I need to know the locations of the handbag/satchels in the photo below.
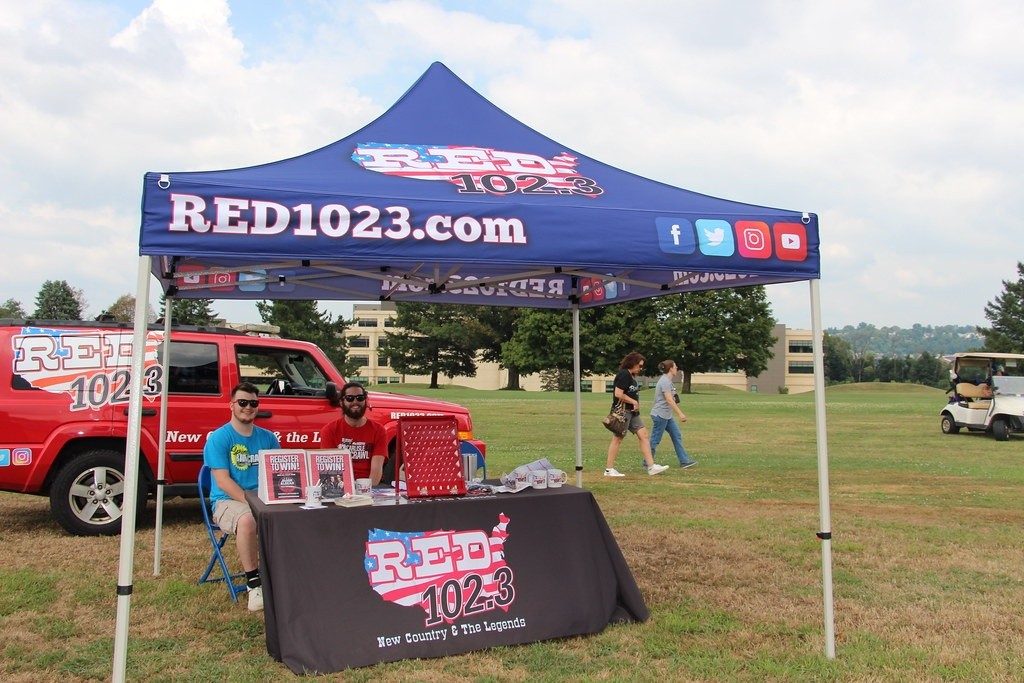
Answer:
[674,394,681,404]
[602,394,633,438]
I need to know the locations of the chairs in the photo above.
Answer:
[458,439,486,482]
[956,383,991,408]
[198,462,263,603]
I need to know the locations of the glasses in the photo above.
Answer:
[233,399,259,408]
[638,364,643,368]
[343,395,366,402]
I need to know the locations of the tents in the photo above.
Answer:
[112,60,836,683]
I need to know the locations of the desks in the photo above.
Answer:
[246,479,650,676]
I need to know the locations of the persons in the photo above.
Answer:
[203,384,281,612]
[327,474,344,492]
[604,352,669,476]
[320,382,390,487]
[642,360,698,469]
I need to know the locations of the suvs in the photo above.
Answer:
[0,318,488,538]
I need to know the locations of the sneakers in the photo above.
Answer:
[648,464,669,476]
[604,469,625,477]
[247,585,264,611]
[681,461,698,469]
[643,465,648,468]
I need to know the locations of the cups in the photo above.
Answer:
[548,469,567,488]
[304,486,322,507]
[355,478,372,496]
[462,453,477,483]
[527,469,547,489]
